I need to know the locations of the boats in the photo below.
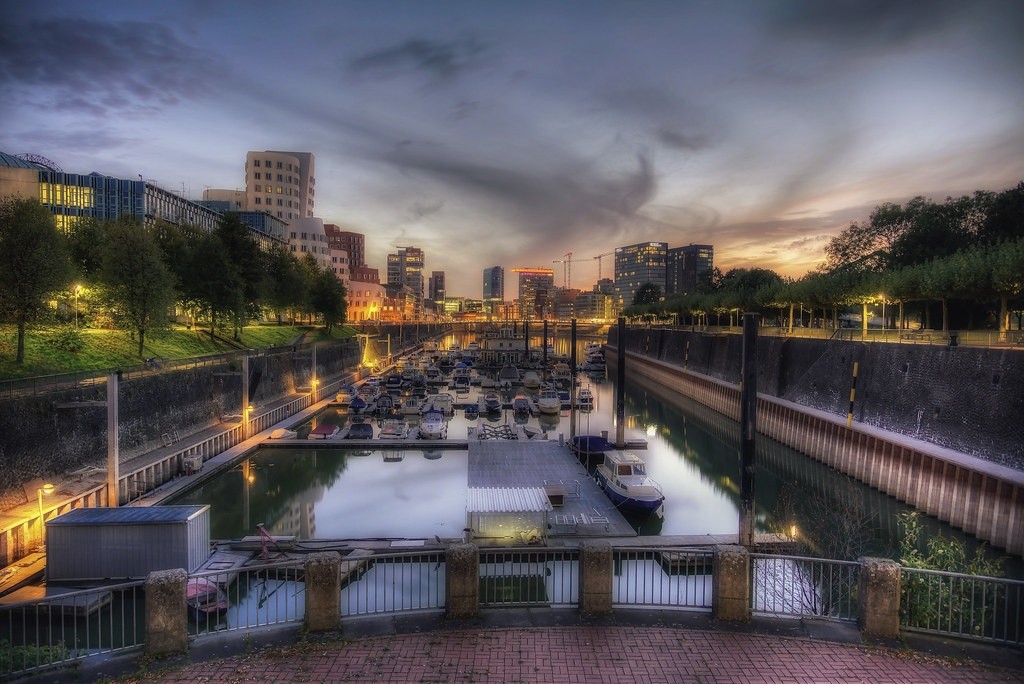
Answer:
[567,435,615,464]
[583,352,607,371]
[307,424,340,439]
[594,449,664,516]
[334,342,594,415]
[417,410,448,439]
[378,420,408,440]
[348,423,374,438]
[270,428,297,440]
[583,344,601,356]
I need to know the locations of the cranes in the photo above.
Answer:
[552,252,595,290]
[593,250,615,280]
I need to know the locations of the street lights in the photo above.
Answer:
[74,284,82,330]
[879,296,885,337]
